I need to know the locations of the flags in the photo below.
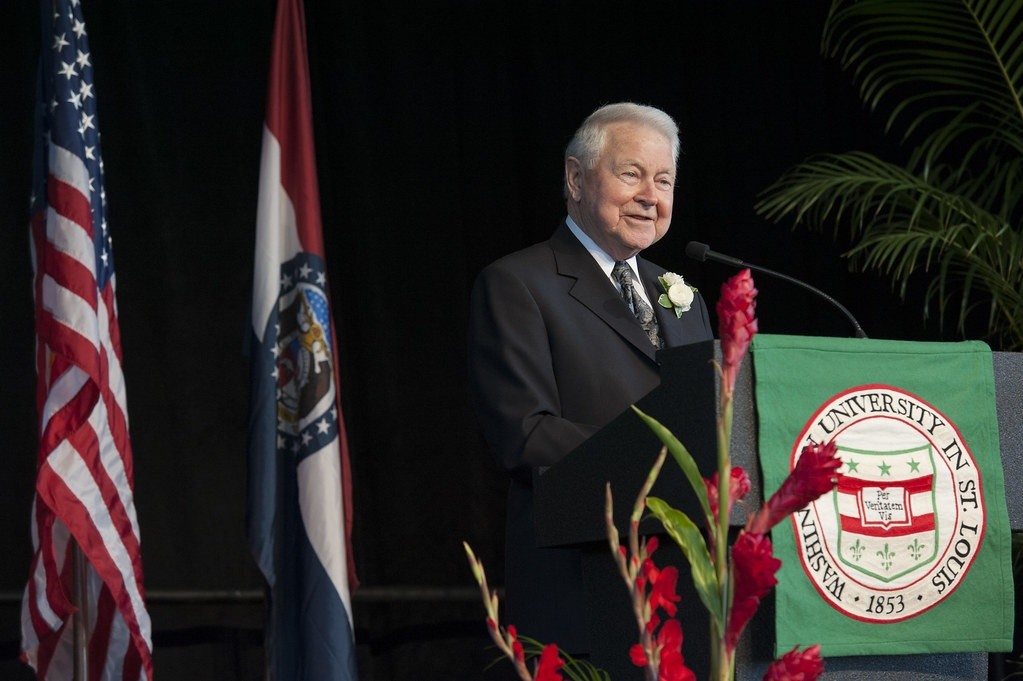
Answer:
[240,0,359,680]
[20,0,153,680]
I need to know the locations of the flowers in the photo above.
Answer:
[461,269,844,681]
[655,271,700,318]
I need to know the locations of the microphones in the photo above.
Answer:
[683,241,870,339]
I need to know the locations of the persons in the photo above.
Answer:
[464,102,714,655]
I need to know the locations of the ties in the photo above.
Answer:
[610,259,666,351]
